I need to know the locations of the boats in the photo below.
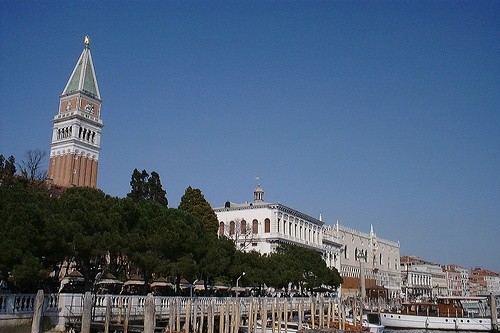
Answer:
[381,301,500,332]
[240,320,385,333]
